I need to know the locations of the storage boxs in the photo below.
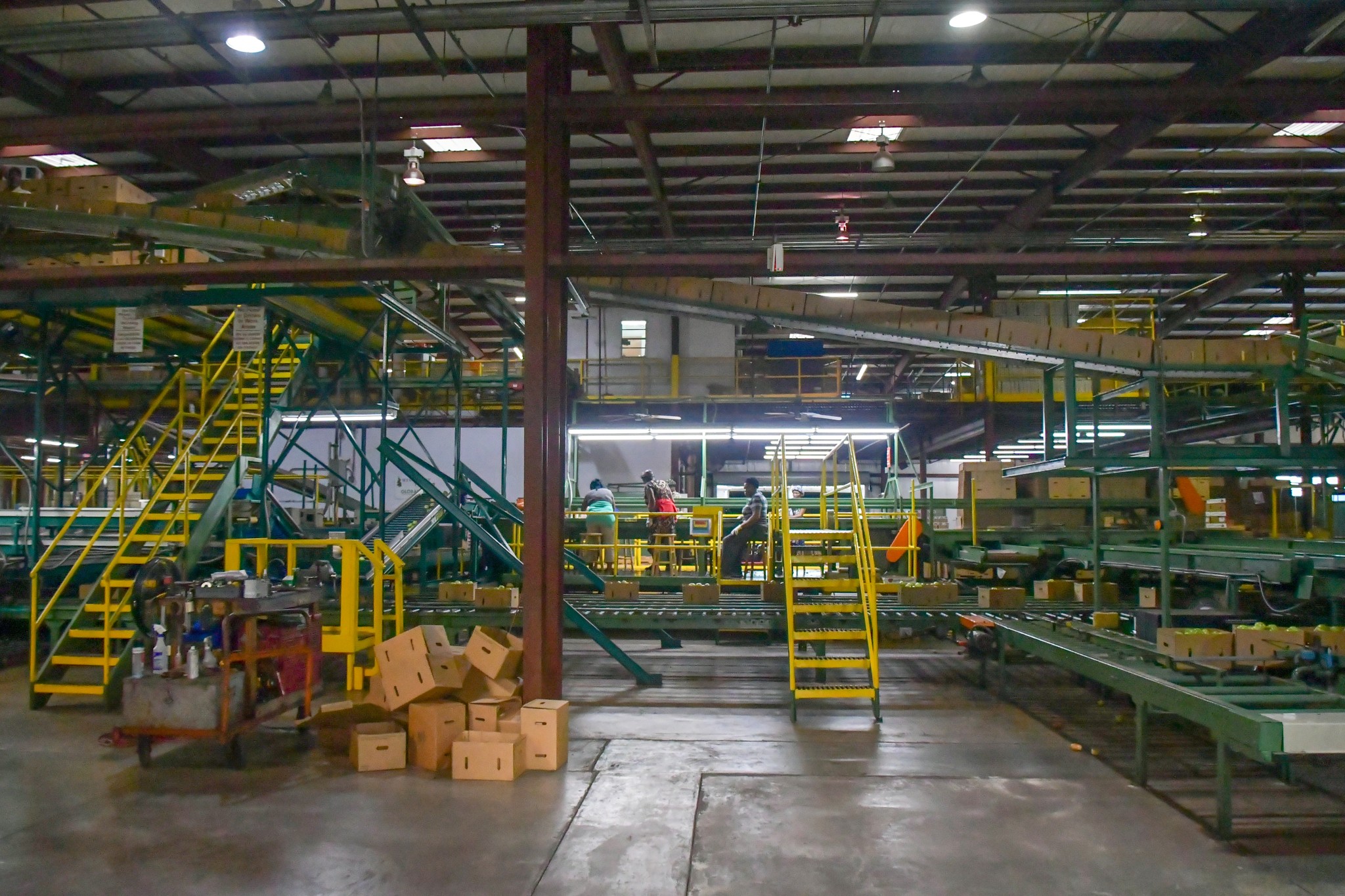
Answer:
[438,580,477,602]
[1299,626,1345,656]
[682,582,719,604]
[761,581,797,602]
[1233,625,1304,666]
[474,586,519,612]
[955,461,1225,532]
[421,238,1155,401]
[891,577,1190,628]
[319,624,568,780]
[124,670,247,726]
[1154,335,1292,381]
[604,580,640,602]
[1157,625,1233,670]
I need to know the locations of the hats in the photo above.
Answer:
[745,477,759,488]
[792,484,804,496]
[640,470,655,478]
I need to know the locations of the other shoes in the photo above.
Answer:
[716,572,743,579]
[645,564,659,571]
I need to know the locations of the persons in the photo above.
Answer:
[581,478,618,573]
[710,476,769,581]
[640,469,677,575]
[775,484,808,575]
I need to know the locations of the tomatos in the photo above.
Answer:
[770,580,776,584]
[407,490,448,532]
[991,587,997,590]
[1172,622,1345,634]
[610,580,633,584]
[450,579,512,590]
[1070,699,1124,755]
[1041,579,1054,582]
[987,529,996,531]
[899,580,939,587]
[688,583,710,586]
[1066,620,1073,626]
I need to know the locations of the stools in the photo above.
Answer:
[744,538,766,579]
[650,532,678,576]
[615,539,634,571]
[579,532,604,573]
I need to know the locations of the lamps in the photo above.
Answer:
[872,128,896,172]
[402,140,426,186]
[835,200,850,239]
[1187,196,1209,236]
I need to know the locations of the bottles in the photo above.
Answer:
[132,647,146,679]
[186,646,200,679]
